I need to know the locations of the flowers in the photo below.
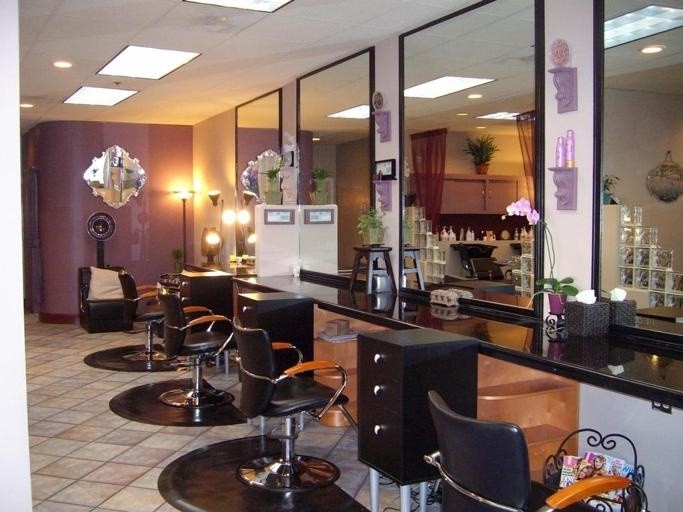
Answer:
[499,196,579,299]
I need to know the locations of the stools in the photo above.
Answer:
[402,246,425,291]
[348,244,390,298]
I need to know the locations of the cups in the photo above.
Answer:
[264,191,282,205]
[486,229,494,241]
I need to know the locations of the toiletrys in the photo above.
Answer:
[566,130,575,168]
[434,225,534,242]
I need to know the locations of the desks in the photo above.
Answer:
[176,254,683,413]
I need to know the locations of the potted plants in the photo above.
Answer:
[356,207,387,247]
[600,171,620,206]
[464,132,500,175]
[308,167,333,205]
[262,165,283,205]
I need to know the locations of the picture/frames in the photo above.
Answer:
[263,207,296,227]
[302,207,335,226]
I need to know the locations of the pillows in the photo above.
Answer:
[82,264,126,302]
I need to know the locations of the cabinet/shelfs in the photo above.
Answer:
[235,292,314,436]
[617,204,681,310]
[405,205,447,287]
[355,326,481,512]
[426,173,522,216]
[510,228,534,297]
[177,265,228,374]
[476,350,583,490]
[308,301,393,429]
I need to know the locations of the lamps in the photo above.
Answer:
[200,226,223,268]
[239,188,256,208]
[169,178,195,263]
[205,187,232,269]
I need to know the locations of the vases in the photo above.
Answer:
[545,291,569,316]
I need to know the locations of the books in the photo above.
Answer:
[316,329,359,343]
[558,451,634,511]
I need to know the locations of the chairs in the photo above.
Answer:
[467,256,509,279]
[117,268,178,362]
[151,281,236,413]
[75,266,130,335]
[226,314,357,495]
[420,389,651,511]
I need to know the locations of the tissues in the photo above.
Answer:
[564,289,610,338]
[600,288,637,327]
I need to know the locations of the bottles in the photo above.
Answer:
[513,226,518,240]
[564,129,574,168]
[519,226,533,240]
[440,225,485,240]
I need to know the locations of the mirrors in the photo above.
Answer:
[397,0,549,321]
[594,0,682,352]
[295,44,377,292]
[232,83,285,271]
[80,143,149,210]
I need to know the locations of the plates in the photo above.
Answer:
[501,230,509,239]
